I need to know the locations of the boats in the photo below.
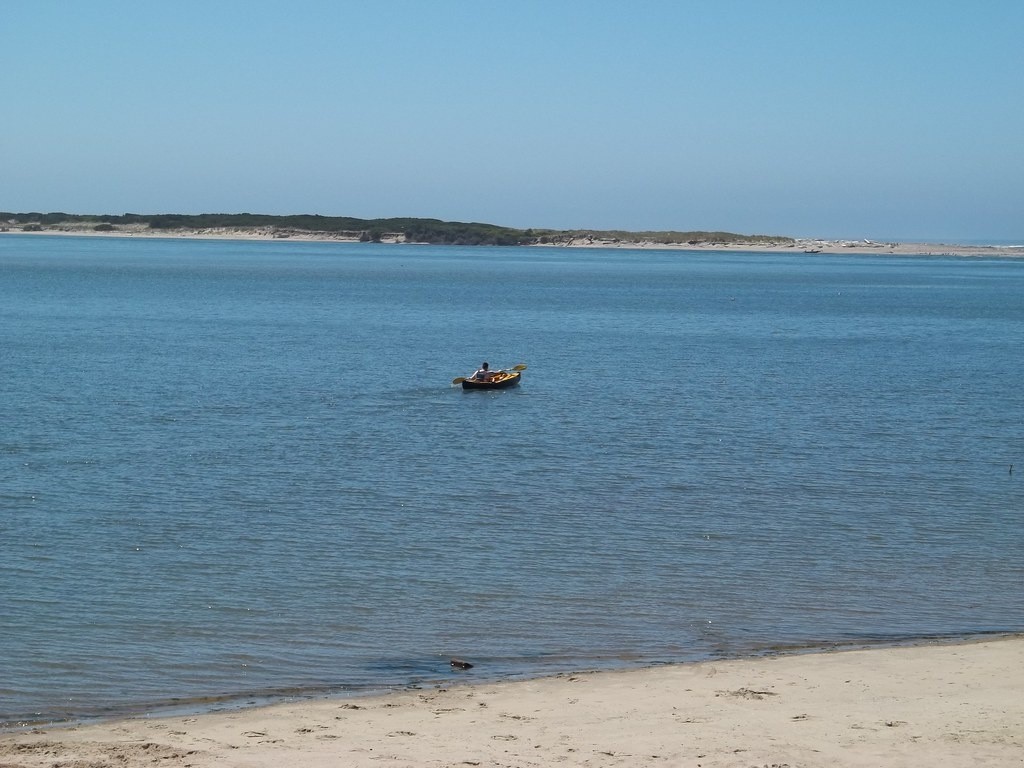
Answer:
[461,371,522,391]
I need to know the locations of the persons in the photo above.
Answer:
[467,362,504,382]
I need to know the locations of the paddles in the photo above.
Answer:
[452,364,527,385]
